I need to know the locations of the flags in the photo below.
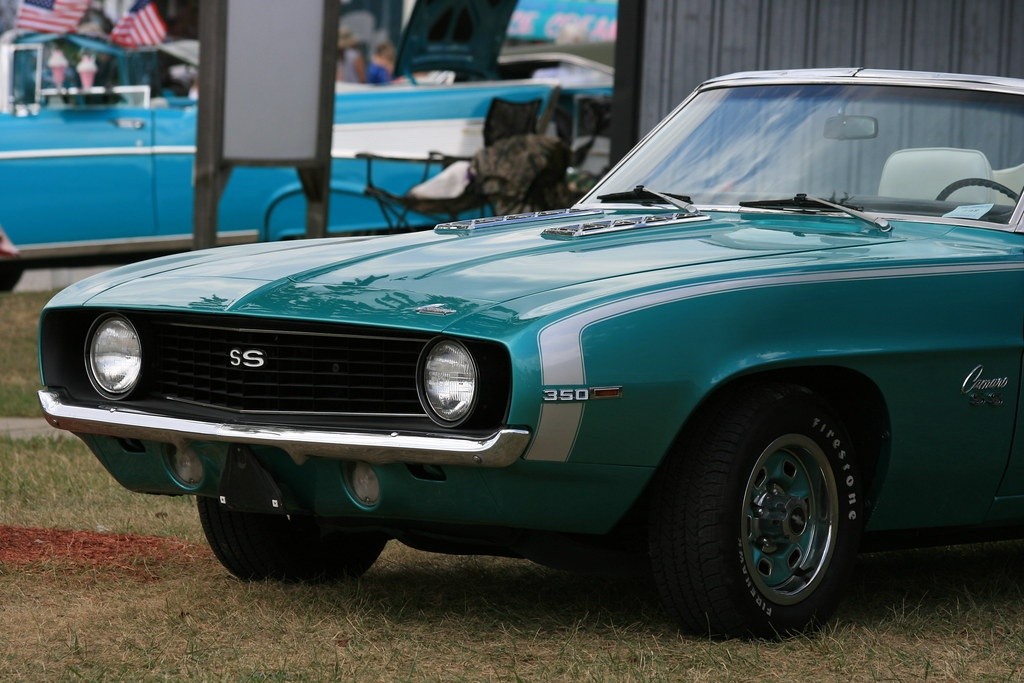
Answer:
[13,0,168,49]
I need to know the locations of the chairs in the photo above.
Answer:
[879,147,996,205]
[354,84,618,232]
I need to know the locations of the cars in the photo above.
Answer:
[35,64,1024,638]
[0,0,615,290]
